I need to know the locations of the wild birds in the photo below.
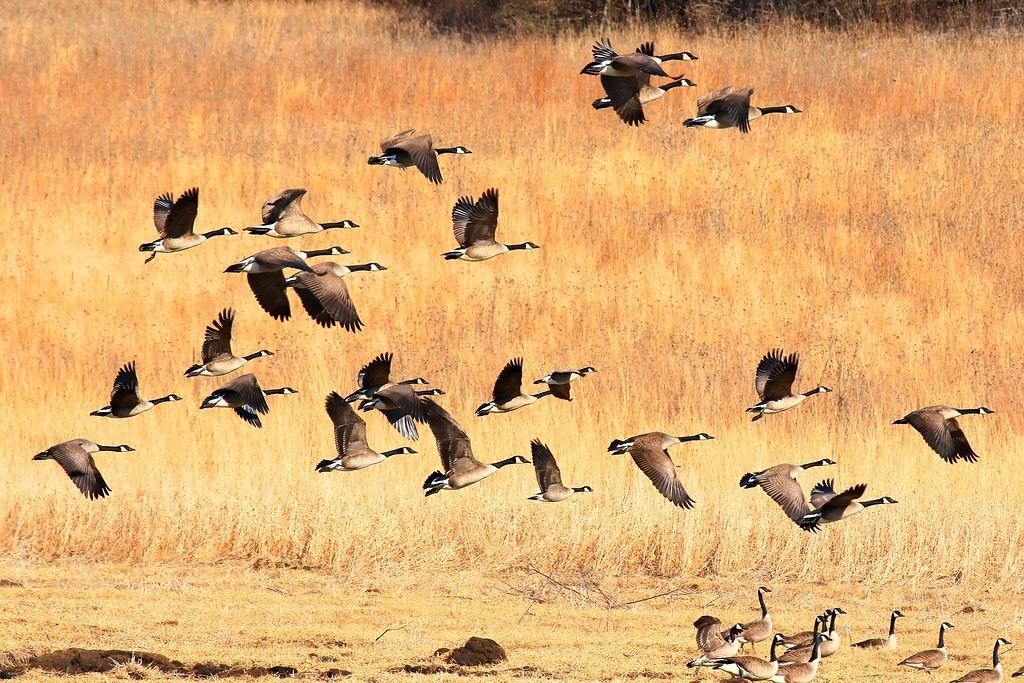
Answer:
[607,433,716,510]
[242,188,361,237]
[313,390,418,473]
[475,357,598,416]
[286,262,387,333]
[744,347,835,424]
[31,438,136,501]
[682,87,802,134]
[739,405,994,534]
[138,186,238,264]
[579,36,699,126]
[342,350,532,497]
[366,130,472,185]
[199,373,299,428]
[182,307,275,378]
[89,361,183,418]
[686,586,1024,683]
[438,188,541,262]
[528,438,594,502]
[223,245,352,322]
